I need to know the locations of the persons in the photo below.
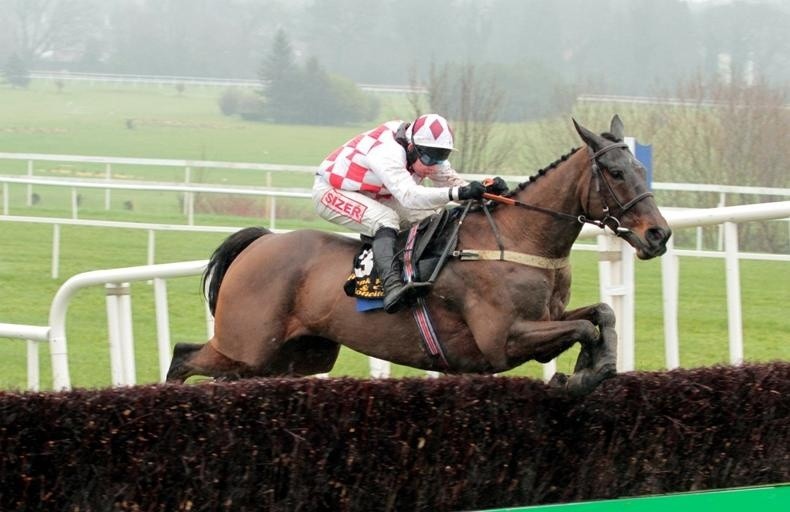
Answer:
[307,113,508,313]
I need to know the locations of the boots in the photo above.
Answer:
[370,229,434,315]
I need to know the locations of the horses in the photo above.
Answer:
[165,114,673,383]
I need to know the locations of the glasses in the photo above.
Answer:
[411,118,446,166]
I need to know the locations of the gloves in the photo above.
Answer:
[457,179,487,202]
[481,177,510,195]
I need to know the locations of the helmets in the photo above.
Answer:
[403,114,463,152]
